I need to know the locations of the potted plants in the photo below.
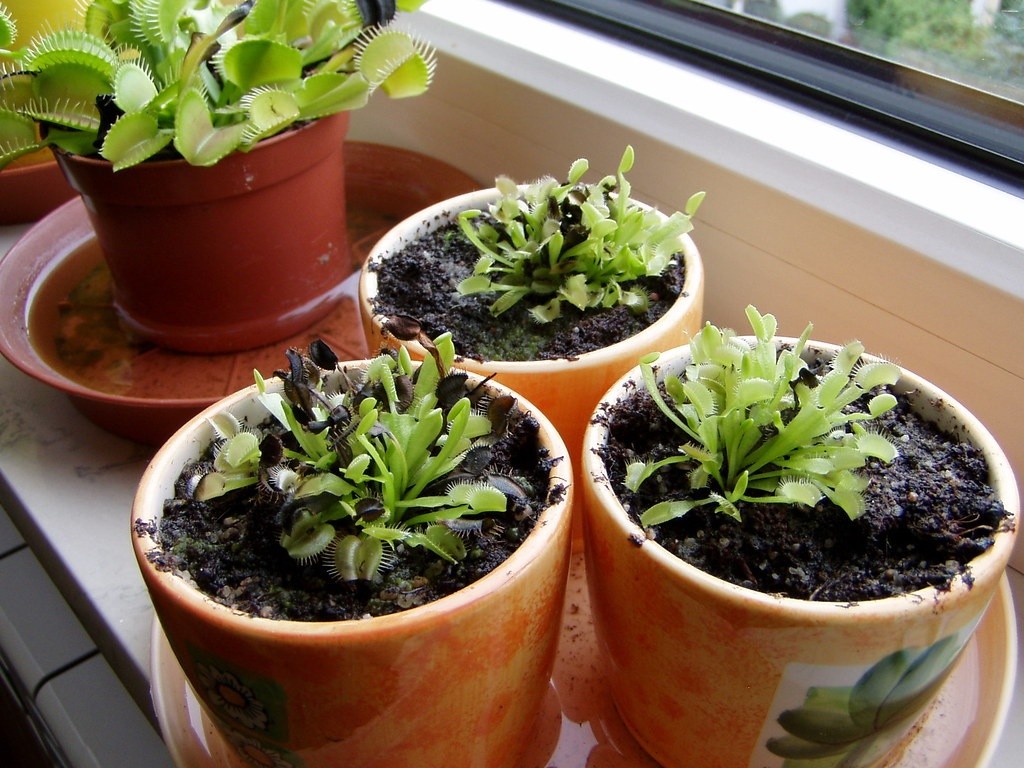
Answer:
[0,0,438,354]
[130,330,575,768]
[581,302,1021,768]
[359,144,706,541]
